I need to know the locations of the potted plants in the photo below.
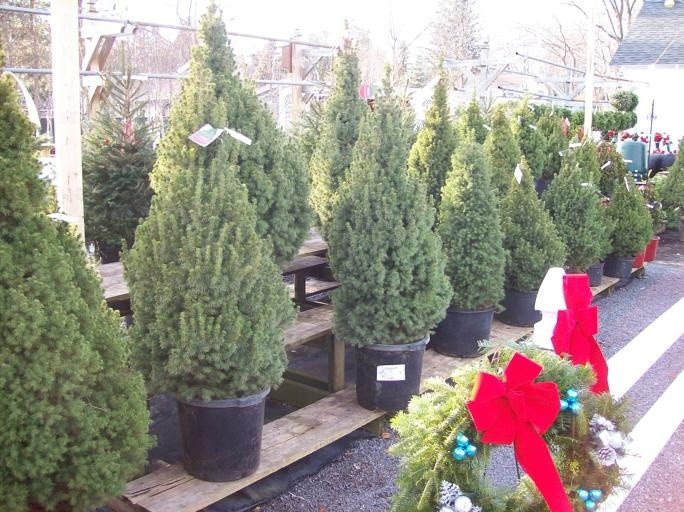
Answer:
[127,71,299,485]
[328,78,450,415]
[436,107,677,360]
